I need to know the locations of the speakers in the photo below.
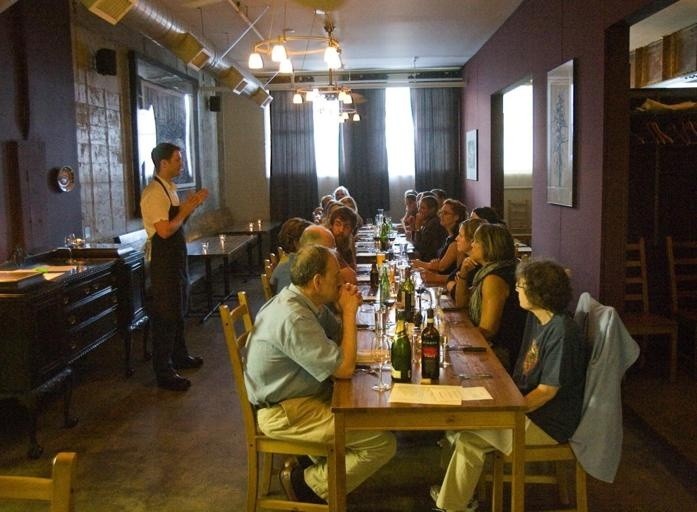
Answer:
[210,96,220,111]
[96,48,117,75]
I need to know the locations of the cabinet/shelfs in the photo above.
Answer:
[0,239,153,462]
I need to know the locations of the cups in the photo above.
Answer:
[219,233,227,245]
[200,241,209,255]
[248,218,263,231]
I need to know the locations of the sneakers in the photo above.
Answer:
[177,353,203,367]
[159,371,191,390]
[427,482,484,510]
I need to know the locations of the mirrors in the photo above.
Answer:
[126,47,203,220]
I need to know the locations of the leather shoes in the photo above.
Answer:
[278,456,302,512]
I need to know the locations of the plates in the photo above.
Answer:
[57,166,76,193]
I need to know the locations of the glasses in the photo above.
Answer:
[436,208,459,218]
[514,279,529,292]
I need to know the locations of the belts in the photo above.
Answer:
[251,399,290,412]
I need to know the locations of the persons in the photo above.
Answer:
[139,142,209,392]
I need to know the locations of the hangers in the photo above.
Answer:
[627,106,696,150]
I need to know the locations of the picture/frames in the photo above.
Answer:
[464,129,479,182]
[544,56,579,210]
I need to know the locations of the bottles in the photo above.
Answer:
[371,209,441,389]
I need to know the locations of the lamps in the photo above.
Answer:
[245,0,361,126]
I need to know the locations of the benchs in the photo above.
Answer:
[112,205,265,326]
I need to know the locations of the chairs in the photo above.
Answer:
[219,291,336,512]
[262,246,287,302]
[505,199,531,250]
[617,233,680,387]
[0,451,79,512]
[663,235,696,375]
[478,292,616,512]
